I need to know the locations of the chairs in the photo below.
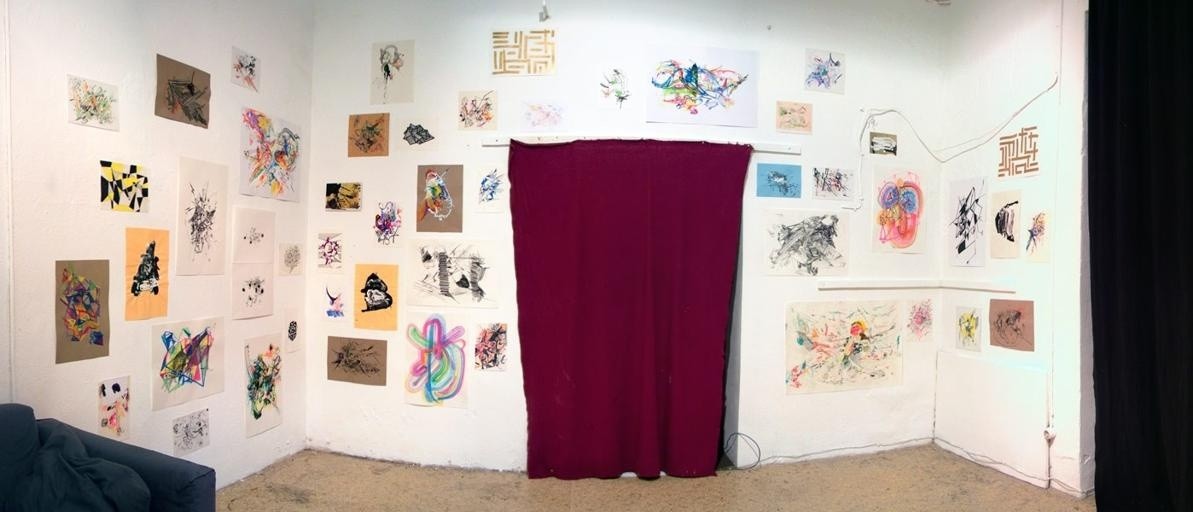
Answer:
[0,401,216,511]
